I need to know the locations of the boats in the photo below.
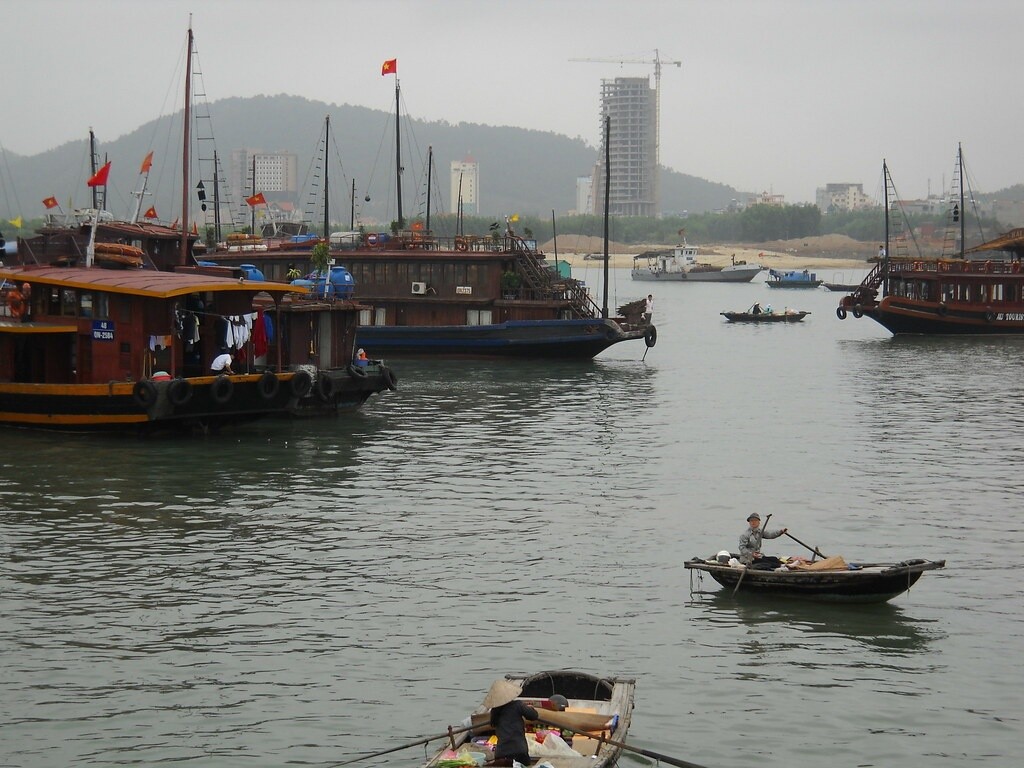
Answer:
[836,228,1024,332]
[0,220,396,435]
[682,551,945,604]
[719,309,811,323]
[825,282,865,290]
[631,245,765,282]
[766,267,820,289]
[186,230,659,358]
[418,668,636,768]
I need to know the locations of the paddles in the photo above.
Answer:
[759,306,765,312]
[537,717,709,768]
[747,301,757,313]
[324,720,491,768]
[732,514,772,600]
[784,528,827,559]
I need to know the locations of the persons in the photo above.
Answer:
[210,348,237,375]
[287,262,302,279]
[738,512,788,568]
[753,302,762,314]
[644,294,654,323]
[0,232,7,263]
[150,344,172,376]
[765,303,775,314]
[878,245,885,256]
[490,681,538,767]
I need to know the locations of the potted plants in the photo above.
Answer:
[286,268,301,304]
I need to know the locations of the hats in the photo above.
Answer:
[747,513,761,523]
[483,679,523,708]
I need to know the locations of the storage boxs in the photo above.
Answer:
[352,359,368,367]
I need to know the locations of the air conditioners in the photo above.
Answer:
[411,282,427,294]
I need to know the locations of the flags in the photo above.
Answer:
[381,58,397,76]
[87,162,111,186]
[43,197,58,209]
[509,213,519,223]
[9,217,21,228]
[411,223,423,230]
[144,207,159,219]
[191,221,199,234]
[139,153,153,174]
[244,192,266,206]
[679,228,685,236]
[171,217,178,230]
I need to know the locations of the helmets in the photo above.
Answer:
[717,550,731,564]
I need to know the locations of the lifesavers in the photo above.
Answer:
[454,239,468,251]
[836,304,864,320]
[347,364,398,391]
[656,273,659,277]
[606,326,657,347]
[6,292,26,317]
[258,371,333,404]
[682,273,686,278]
[937,304,997,322]
[132,377,234,407]
[912,261,1022,275]
[365,233,380,247]
[226,234,264,245]
[92,243,144,265]
